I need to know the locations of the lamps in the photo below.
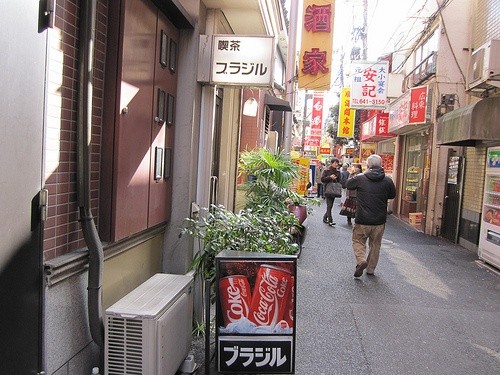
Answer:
[244,86,258,117]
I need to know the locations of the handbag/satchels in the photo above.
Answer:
[325,179,342,198]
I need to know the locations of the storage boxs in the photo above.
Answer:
[408,212,422,225]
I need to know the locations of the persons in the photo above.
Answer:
[316,160,362,226]
[346,155,397,277]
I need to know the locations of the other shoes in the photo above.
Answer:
[366,270,374,275]
[354,261,368,277]
[323,218,329,223]
[329,221,336,225]
[348,219,352,225]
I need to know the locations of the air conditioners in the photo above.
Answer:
[103,272,195,375]
[466,39,500,92]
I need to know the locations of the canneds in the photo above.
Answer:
[284,276,294,328]
[248,264,291,327]
[219,275,252,328]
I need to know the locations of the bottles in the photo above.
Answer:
[486,176,500,208]
